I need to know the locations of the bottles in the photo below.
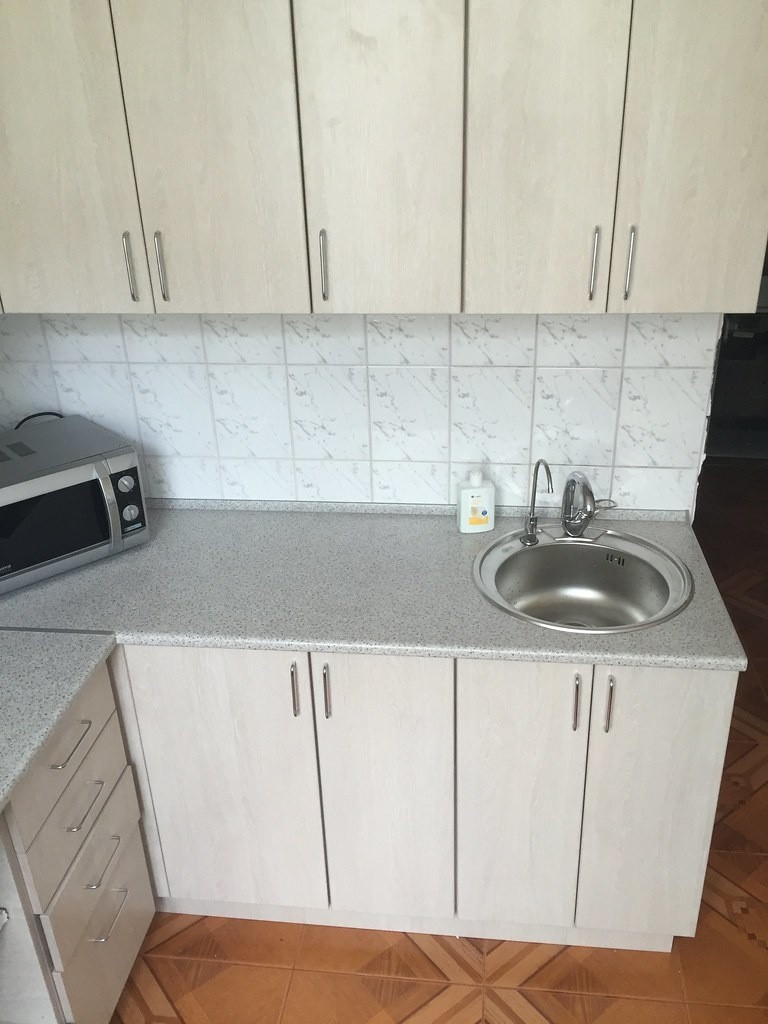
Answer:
[457,469,496,533]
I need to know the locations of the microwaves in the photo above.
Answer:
[0,414,150,594]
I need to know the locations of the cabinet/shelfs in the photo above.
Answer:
[462,0,768,315]
[291,0,466,316]
[456,657,740,953]
[0,0,315,316]
[122,643,458,936]
[0,663,156,1024]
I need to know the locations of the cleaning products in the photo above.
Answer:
[455,469,496,534]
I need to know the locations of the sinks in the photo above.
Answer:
[472,518,696,635]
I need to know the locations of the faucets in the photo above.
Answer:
[561,472,596,538]
[526,458,553,534]
